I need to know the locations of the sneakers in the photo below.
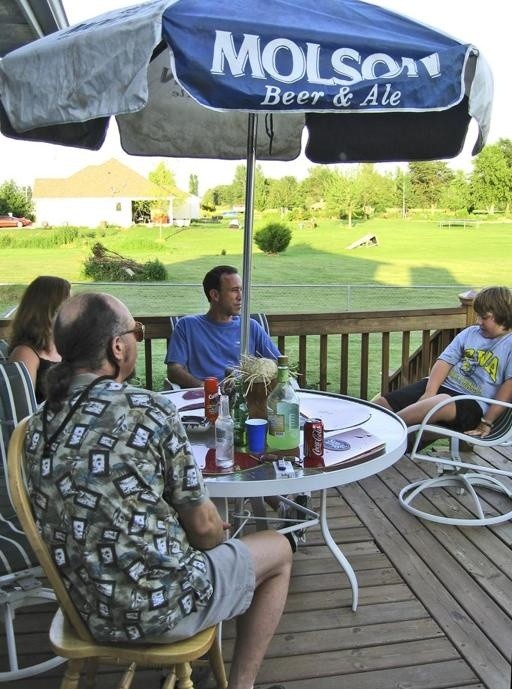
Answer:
[275,525,307,552]
[286,491,311,528]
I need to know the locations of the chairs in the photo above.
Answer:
[163,313,299,393]
[398,380,512,526]
[5,413,226,689]
[1,336,71,682]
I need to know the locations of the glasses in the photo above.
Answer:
[119,321,147,343]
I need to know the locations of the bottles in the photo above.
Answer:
[213,394,236,467]
[262,353,300,451]
[230,379,252,453]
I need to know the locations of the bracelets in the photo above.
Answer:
[480,418,493,428]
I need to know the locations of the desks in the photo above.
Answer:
[159,387,410,652]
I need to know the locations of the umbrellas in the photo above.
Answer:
[0,0,494,530]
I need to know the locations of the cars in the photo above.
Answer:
[0,216,33,228]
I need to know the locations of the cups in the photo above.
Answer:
[245,419,269,454]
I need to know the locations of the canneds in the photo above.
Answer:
[204,377,218,422]
[303,419,324,459]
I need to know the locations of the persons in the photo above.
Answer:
[164,265,310,522]
[369,286,512,447]
[9,273,72,406]
[22,292,293,686]
